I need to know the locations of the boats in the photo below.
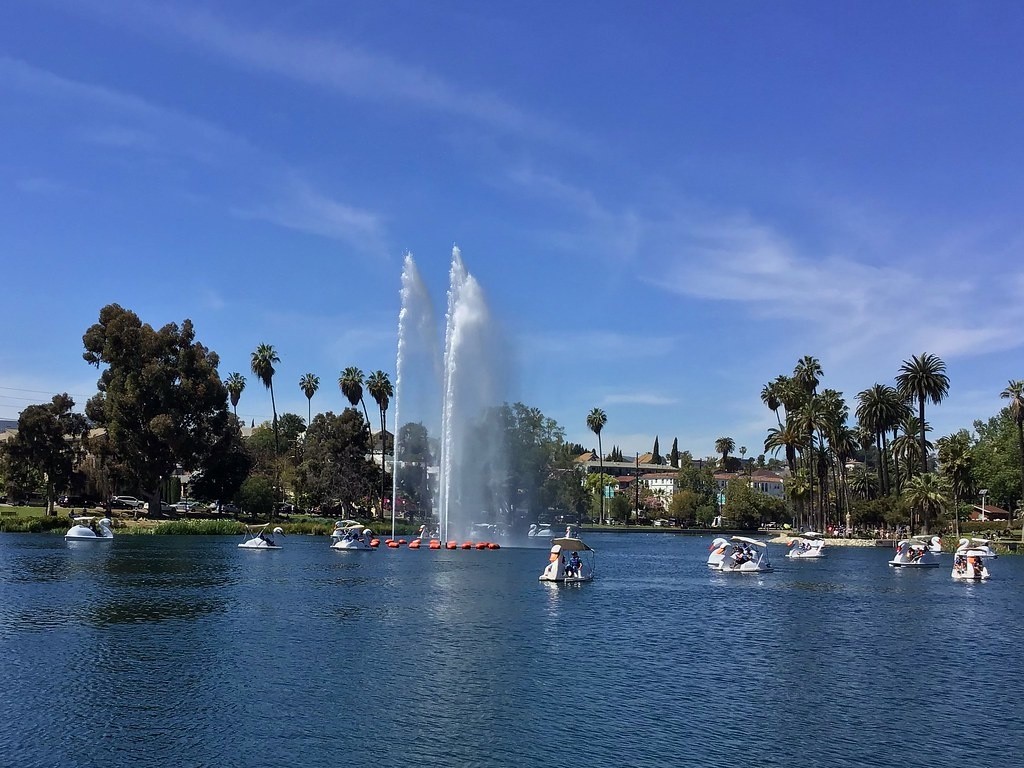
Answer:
[330,519,379,550]
[888,534,1000,579]
[528,522,557,537]
[64,515,114,539]
[237,522,283,549]
[416,520,454,539]
[707,535,775,572]
[785,531,829,558]
[538,537,596,581]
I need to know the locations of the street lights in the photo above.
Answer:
[978,488,988,522]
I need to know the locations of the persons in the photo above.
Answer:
[906,545,929,563]
[572,528,578,539]
[70,502,112,537]
[977,528,1011,540]
[953,555,983,576]
[729,542,753,569]
[800,523,907,540]
[343,529,359,541]
[938,531,942,538]
[958,524,963,536]
[260,533,274,545]
[561,551,583,578]
[800,542,811,554]
[64,495,69,508]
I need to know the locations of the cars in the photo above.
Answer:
[0,490,307,517]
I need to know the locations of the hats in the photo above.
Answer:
[572,552,577,555]
